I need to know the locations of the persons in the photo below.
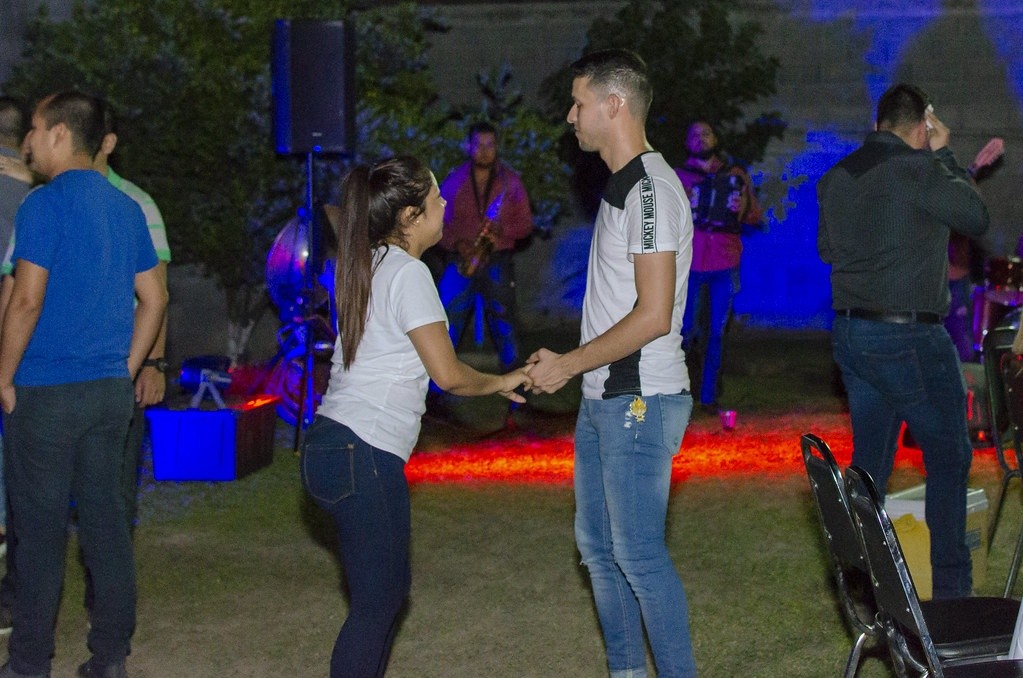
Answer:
[813,80,987,603]
[944,229,980,362]
[302,156,534,678]
[430,121,532,436]
[674,117,762,419]
[525,51,695,678]
[0,89,172,678]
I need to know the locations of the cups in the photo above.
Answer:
[719,410,737,431]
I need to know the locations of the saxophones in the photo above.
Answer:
[456,159,510,282]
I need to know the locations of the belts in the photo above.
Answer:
[838,306,939,325]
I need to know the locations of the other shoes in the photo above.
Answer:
[0,659,49,678]
[78,654,126,678]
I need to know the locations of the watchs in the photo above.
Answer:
[144,358,167,372]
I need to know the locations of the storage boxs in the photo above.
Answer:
[144,394,282,481]
[881,483,988,600]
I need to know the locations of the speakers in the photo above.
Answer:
[271,17,356,163]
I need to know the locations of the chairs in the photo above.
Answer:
[800,308,1023,677]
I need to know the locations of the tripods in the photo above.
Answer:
[254,157,332,458]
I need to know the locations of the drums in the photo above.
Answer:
[982,253,1023,306]
